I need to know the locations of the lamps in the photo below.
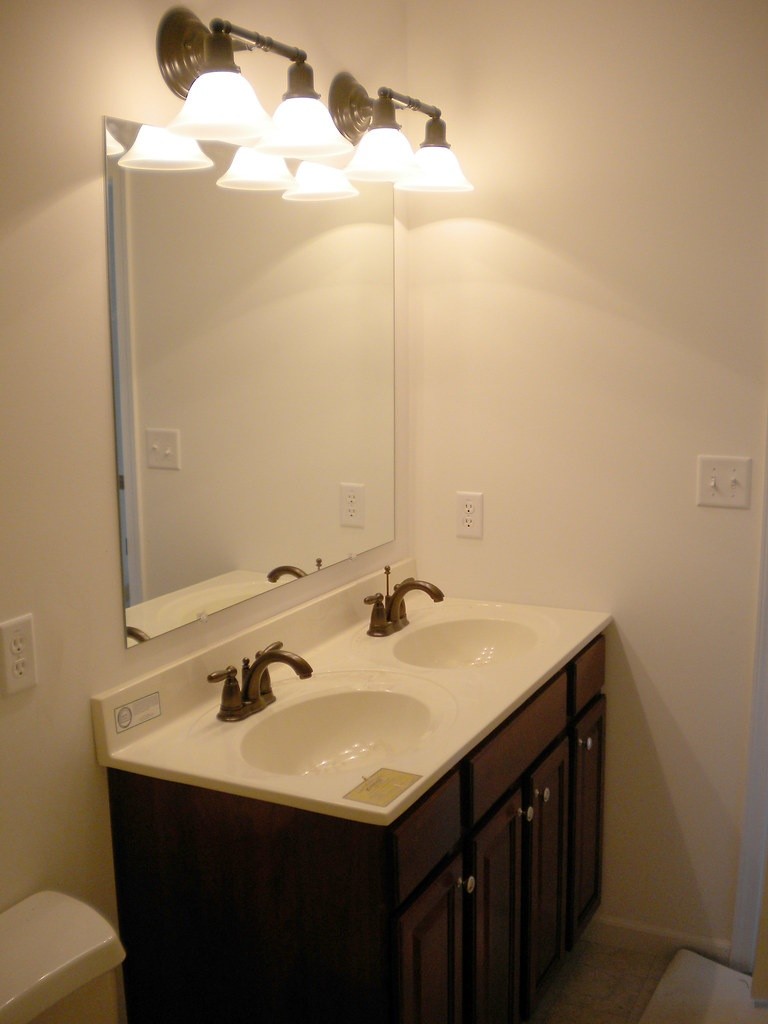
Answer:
[215,146,360,202]
[328,71,475,193]
[154,7,349,156]
[102,117,215,171]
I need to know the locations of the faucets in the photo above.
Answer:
[266,557,324,585]
[237,647,315,713]
[377,579,446,637]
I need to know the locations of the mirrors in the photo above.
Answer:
[99,109,400,652]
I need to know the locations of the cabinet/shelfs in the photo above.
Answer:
[108,630,614,1024]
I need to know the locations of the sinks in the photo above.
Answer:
[390,616,544,673]
[156,584,267,627]
[240,688,442,787]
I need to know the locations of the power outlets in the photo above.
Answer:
[1,613,39,694]
